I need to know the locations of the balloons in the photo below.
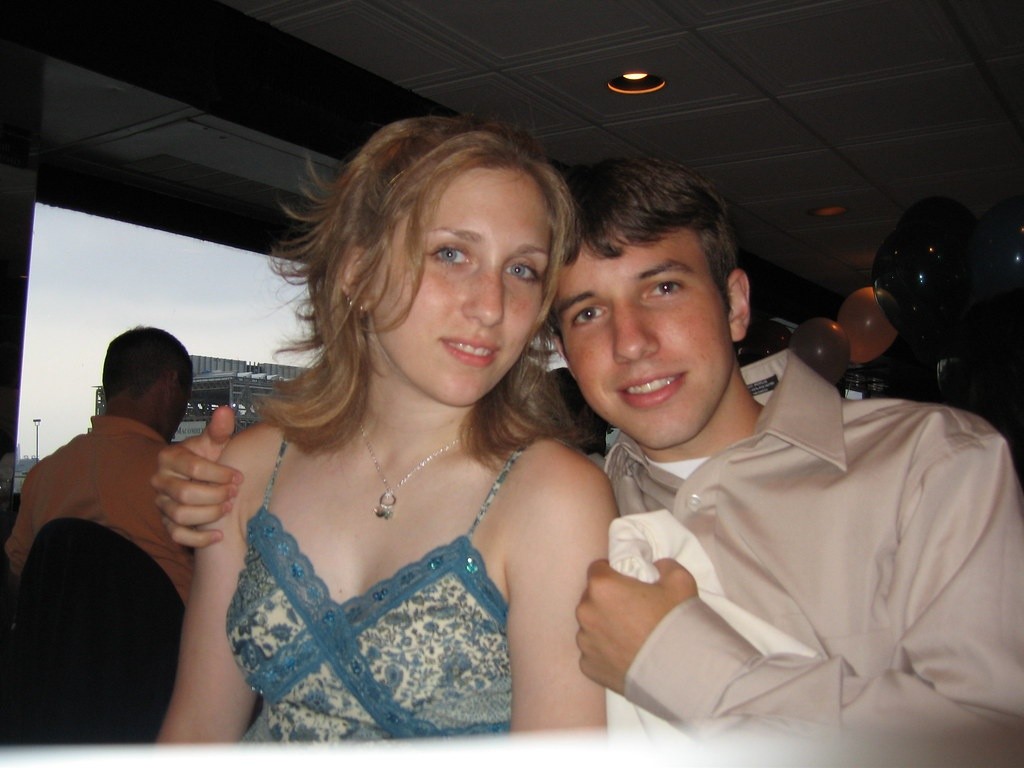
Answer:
[738,195,1024,386]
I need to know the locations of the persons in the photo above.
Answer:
[148,155,1024,757]
[3,328,194,606]
[542,368,612,460]
[156,116,618,745]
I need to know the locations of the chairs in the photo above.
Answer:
[0,516,185,744]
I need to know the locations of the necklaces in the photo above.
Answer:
[359,418,468,520]
